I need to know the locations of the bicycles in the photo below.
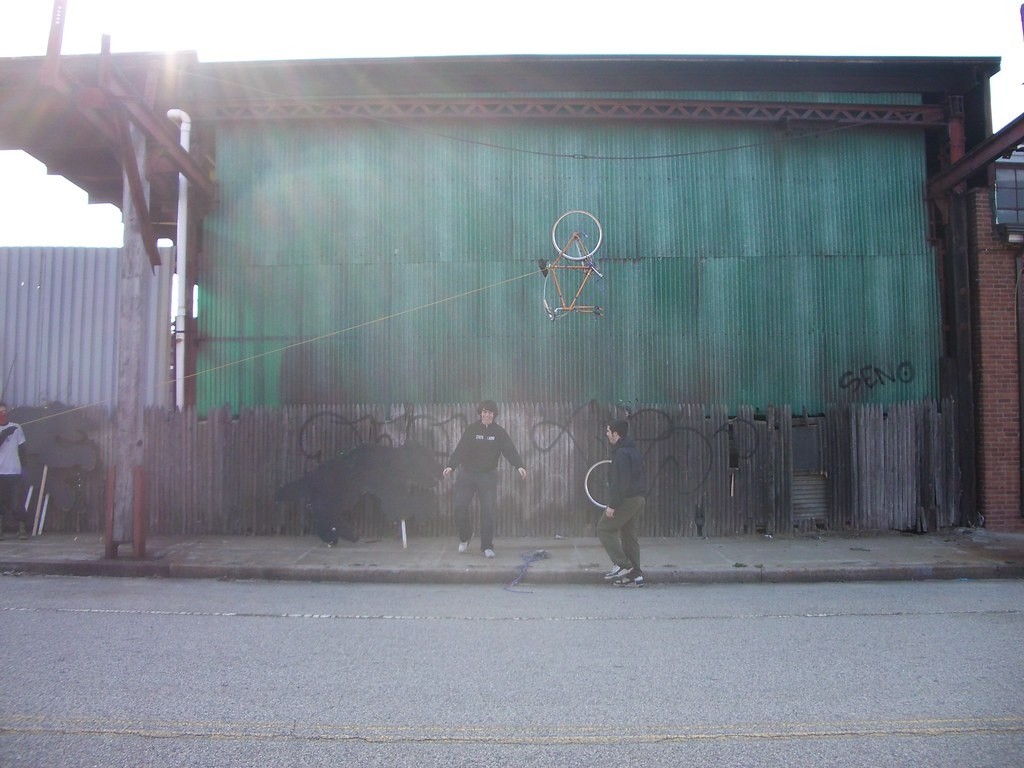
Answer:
[584,459,612,509]
[542,209,607,322]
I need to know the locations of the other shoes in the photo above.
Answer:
[484,549,495,558]
[458,541,469,552]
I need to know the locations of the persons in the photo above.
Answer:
[0,404,27,540]
[443,400,527,557]
[596,419,646,586]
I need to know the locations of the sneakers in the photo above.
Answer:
[614,574,644,587]
[605,564,634,579]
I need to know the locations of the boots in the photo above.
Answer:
[0,519,5,540]
[17,520,28,539]
